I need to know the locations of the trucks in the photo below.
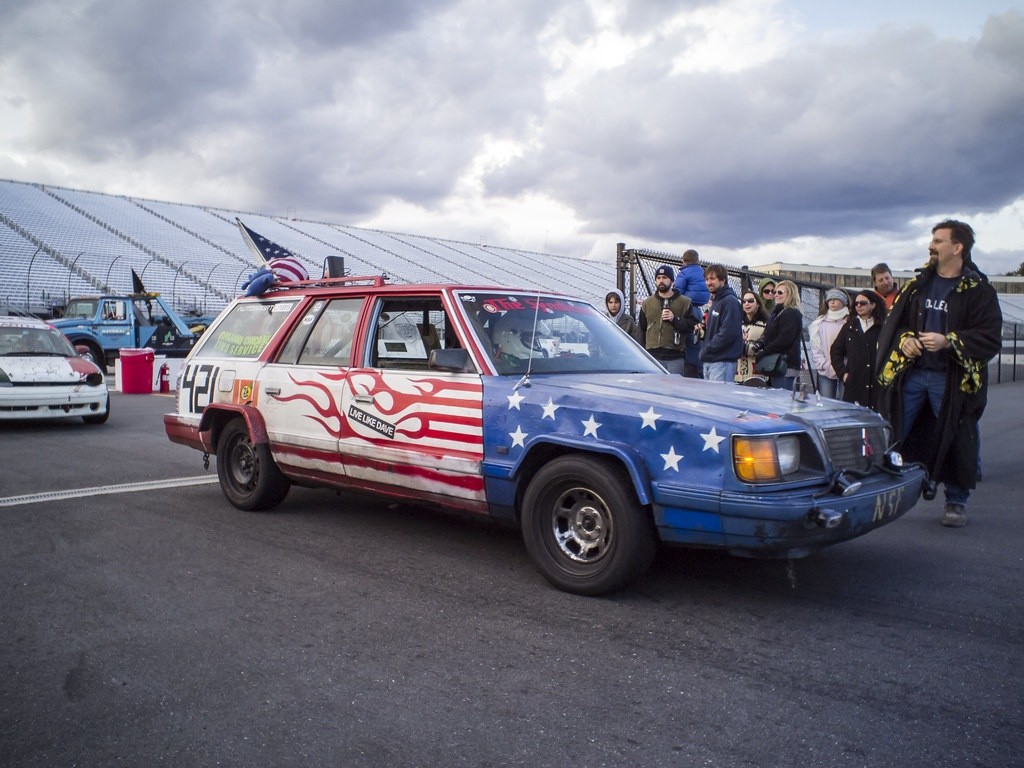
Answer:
[25,292,218,374]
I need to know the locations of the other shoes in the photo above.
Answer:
[941,502,967,526]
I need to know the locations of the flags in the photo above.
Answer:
[132,269,144,293]
[237,221,309,287]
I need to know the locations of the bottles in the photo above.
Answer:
[663,297,670,309]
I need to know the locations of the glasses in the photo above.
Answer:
[742,298,754,303]
[762,289,774,294]
[854,301,872,306]
[774,291,784,294]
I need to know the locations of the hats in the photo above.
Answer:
[655,265,674,282]
[826,287,851,308]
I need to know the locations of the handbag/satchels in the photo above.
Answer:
[752,354,788,377]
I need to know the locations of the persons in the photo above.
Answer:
[673,250,803,391]
[493,310,561,360]
[809,263,899,413]
[877,220,1002,527]
[588,288,637,358]
[636,266,700,378]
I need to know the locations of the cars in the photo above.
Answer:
[0,315,112,427]
[161,275,929,597]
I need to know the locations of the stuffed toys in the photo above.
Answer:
[241,264,276,297]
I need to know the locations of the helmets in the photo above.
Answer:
[492,311,551,359]
